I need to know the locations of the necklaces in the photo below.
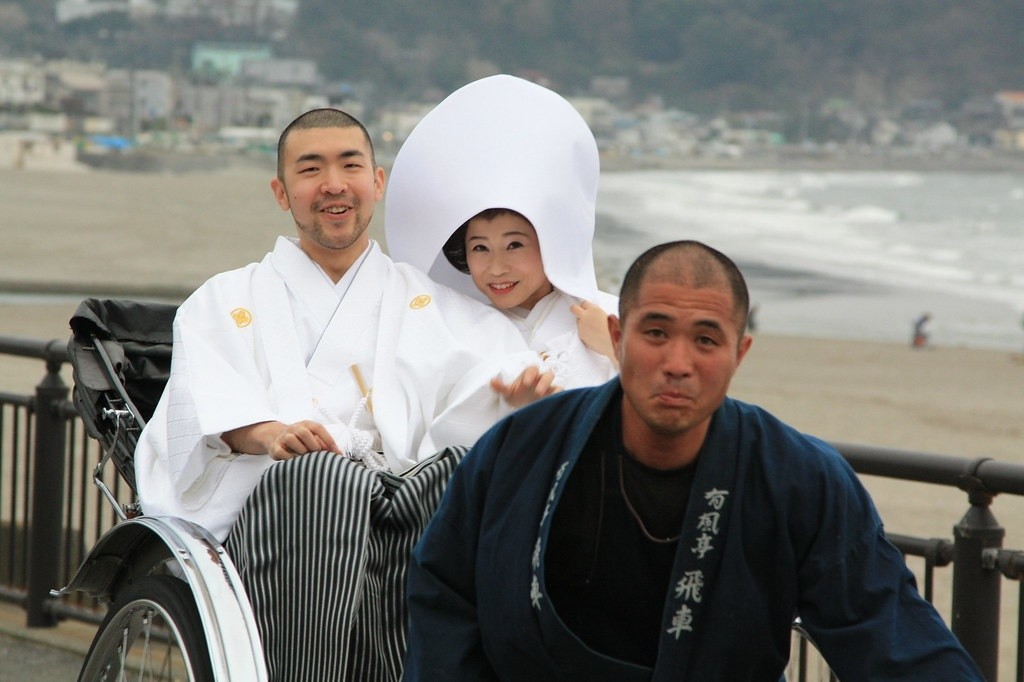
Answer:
[618,454,681,542]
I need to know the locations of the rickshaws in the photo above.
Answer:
[51,297,844,682]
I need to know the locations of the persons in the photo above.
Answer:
[385,74,620,391]
[134,108,566,682]
[401,239,984,682]
